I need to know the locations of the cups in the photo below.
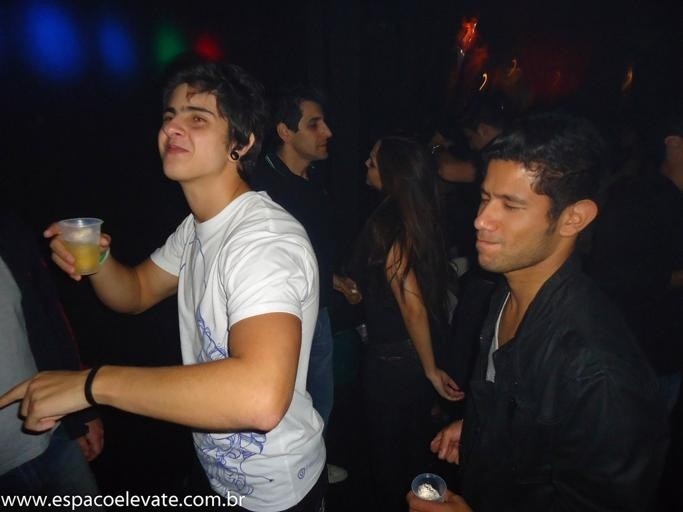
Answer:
[411,472,446,505]
[342,282,363,306]
[58,218,105,276]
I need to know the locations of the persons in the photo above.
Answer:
[1,206,105,509]
[3,49,331,512]
[243,82,680,512]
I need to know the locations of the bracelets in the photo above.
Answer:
[84,364,103,406]
